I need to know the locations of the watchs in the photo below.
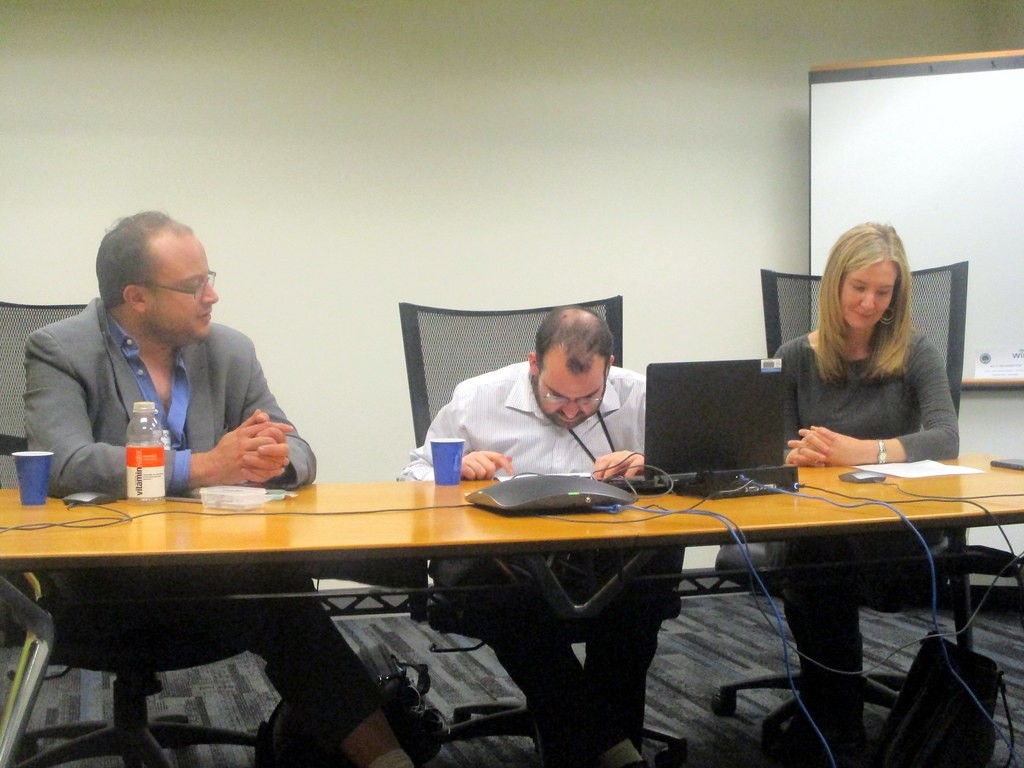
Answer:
[877,439,887,464]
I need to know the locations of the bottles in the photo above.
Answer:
[125,401,165,504]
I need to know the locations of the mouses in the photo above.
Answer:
[63,492,118,507]
[839,472,887,483]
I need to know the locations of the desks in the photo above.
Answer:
[0,455,1023,570]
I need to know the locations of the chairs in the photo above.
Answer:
[713,257,975,718]
[0,303,255,768]
[399,295,690,768]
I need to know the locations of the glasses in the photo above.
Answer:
[537,373,606,406]
[130,270,216,299]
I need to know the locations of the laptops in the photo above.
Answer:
[604,356,787,491]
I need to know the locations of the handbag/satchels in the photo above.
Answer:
[255,645,451,768]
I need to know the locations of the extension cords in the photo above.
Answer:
[696,463,801,500]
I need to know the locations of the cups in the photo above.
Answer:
[429,437,465,485]
[11,452,53,505]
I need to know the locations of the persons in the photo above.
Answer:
[402,307,663,768]
[771,223,959,768]
[23,209,413,768]
